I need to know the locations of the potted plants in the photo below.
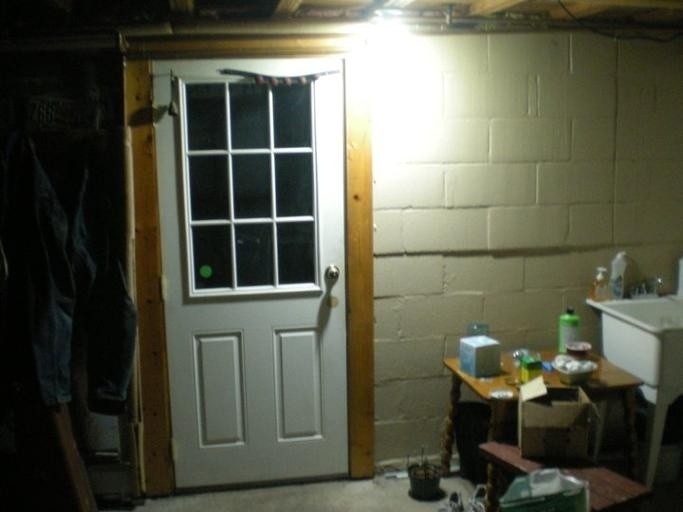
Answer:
[407,447,440,499]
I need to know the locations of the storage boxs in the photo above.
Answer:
[517,375,592,459]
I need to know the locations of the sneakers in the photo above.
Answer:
[465,483,494,510]
[433,490,463,511]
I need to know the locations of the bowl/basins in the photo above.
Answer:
[566,343,592,358]
[552,359,597,385]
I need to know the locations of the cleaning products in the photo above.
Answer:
[590,268,609,302]
[609,251,626,301]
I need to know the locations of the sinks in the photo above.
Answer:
[585,296,683,403]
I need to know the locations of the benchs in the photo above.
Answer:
[478,439,651,511]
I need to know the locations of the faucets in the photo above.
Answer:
[649,276,665,297]
